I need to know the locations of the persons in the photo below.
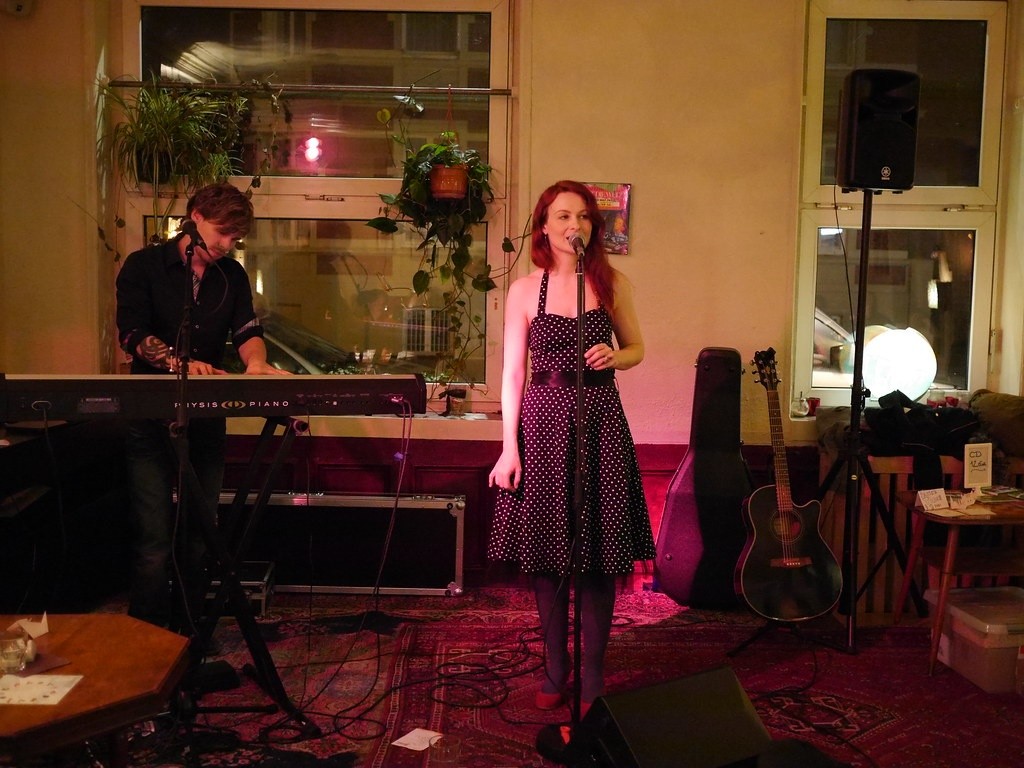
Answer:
[115,182,295,656]
[489,179,657,720]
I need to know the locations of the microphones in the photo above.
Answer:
[179,215,208,251]
[568,233,586,257]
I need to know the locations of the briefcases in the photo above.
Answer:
[203,559,276,615]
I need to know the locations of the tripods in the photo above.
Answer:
[809,188,928,656]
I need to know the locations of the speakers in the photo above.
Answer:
[838,68,922,195]
[572,662,772,768]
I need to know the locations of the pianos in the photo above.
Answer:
[0,369,428,419]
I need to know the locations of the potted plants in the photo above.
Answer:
[365,67,536,415]
[97,66,249,200]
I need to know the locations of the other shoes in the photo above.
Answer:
[536,685,562,710]
[581,700,592,719]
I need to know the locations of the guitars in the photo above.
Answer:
[733,347,843,624]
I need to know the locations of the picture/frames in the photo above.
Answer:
[965,443,992,489]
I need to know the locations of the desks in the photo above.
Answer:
[881,483,1024,678]
[0,613,190,768]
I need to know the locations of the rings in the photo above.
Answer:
[604,356,609,363]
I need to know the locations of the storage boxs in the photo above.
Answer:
[922,586,1024,696]
